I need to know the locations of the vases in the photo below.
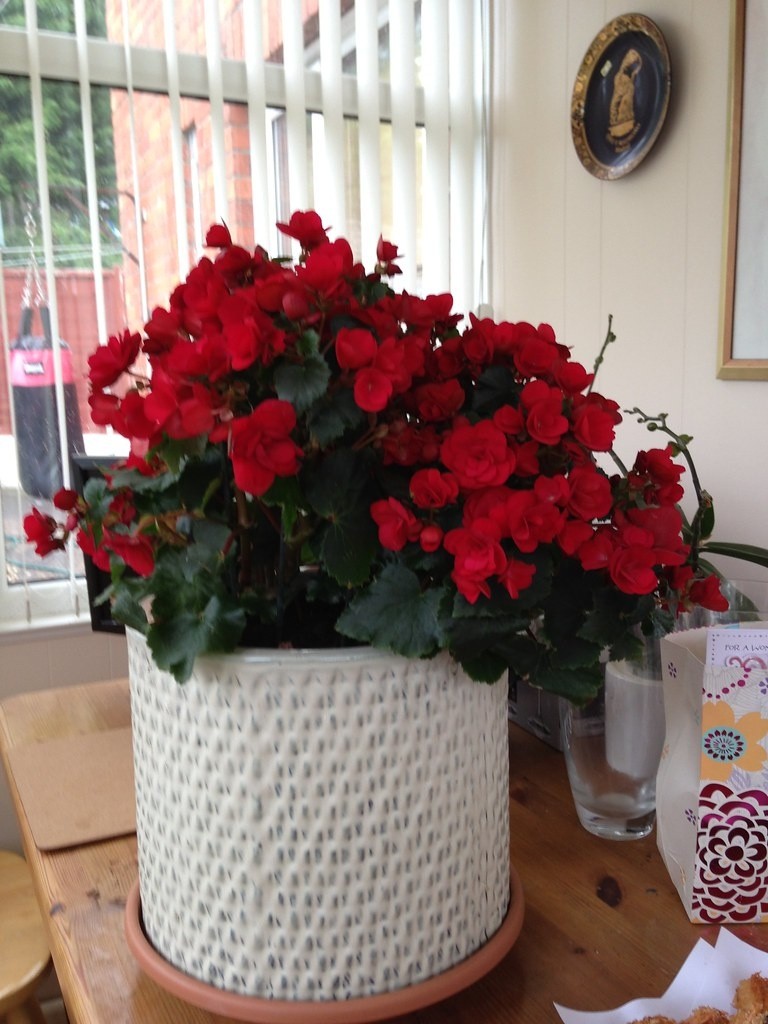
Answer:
[122,634,529,1009]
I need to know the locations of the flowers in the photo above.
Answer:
[22,205,726,701]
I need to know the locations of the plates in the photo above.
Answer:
[569,13,672,181]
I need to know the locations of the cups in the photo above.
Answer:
[665,578,768,633]
[555,630,665,840]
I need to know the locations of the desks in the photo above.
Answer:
[1,620,768,1024]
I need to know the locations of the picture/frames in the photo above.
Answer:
[712,1,767,382]
[566,12,673,181]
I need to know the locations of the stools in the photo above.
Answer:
[0,848,52,1024]
